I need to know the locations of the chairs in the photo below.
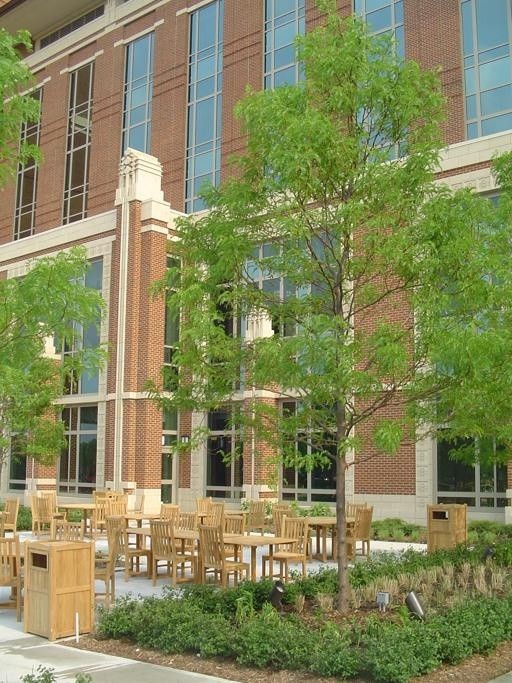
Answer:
[0,489,373,623]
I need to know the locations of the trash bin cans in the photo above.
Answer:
[24,539,95,641]
[427,504,468,552]
